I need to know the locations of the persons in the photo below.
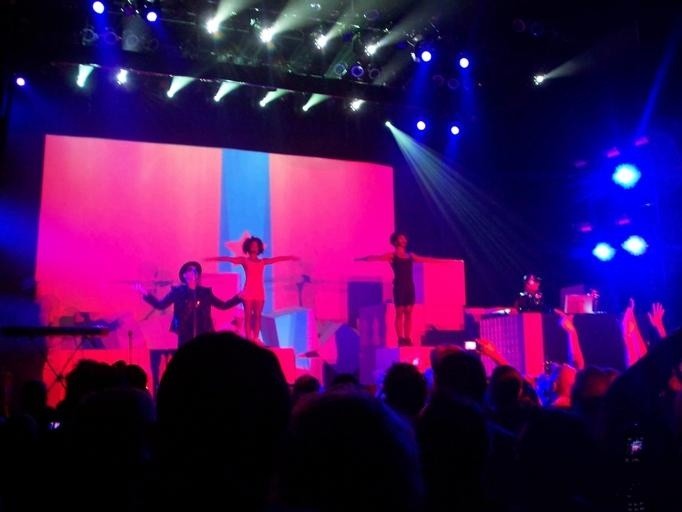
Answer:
[134,261,244,350]
[511,275,567,318]
[17,298,682,511]
[351,230,440,346]
[206,236,299,338]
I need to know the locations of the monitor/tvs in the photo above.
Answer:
[564,295,594,314]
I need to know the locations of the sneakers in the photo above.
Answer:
[398,337,412,346]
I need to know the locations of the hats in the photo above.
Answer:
[179,261,202,282]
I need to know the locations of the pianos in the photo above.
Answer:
[0,325,111,336]
